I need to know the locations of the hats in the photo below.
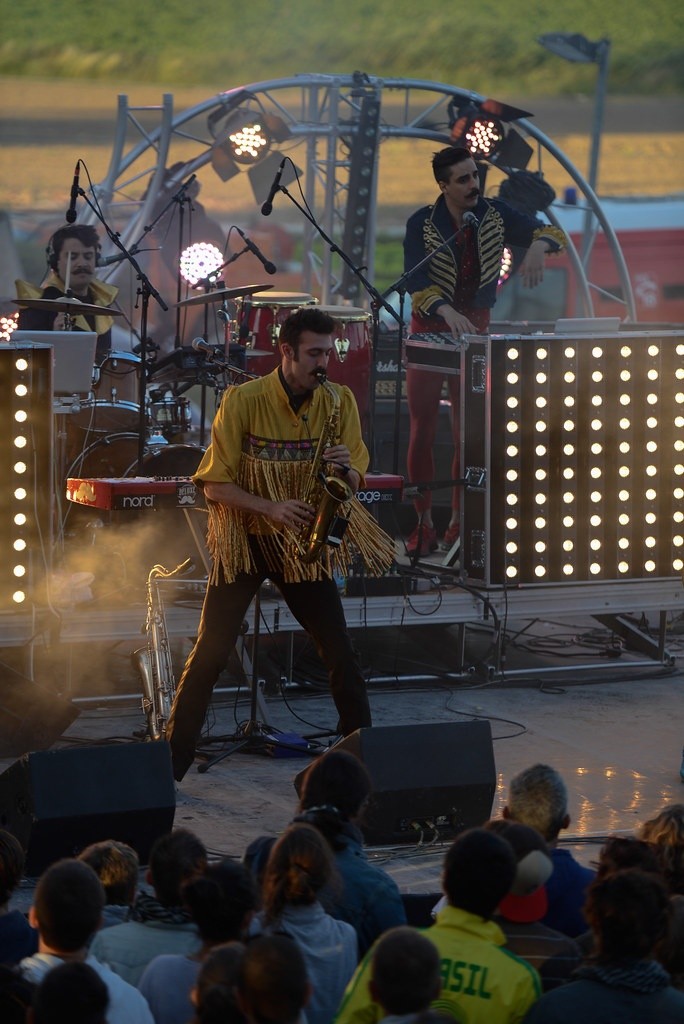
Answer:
[497,825,554,922]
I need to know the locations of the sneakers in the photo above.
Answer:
[441,524,459,551]
[404,524,438,557]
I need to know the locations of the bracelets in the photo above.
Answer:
[533,236,563,253]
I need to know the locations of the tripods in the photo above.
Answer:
[196,590,322,774]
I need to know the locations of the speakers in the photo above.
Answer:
[0,662,84,759]
[0,742,177,877]
[292,719,495,847]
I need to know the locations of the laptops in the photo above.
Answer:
[554,318,620,334]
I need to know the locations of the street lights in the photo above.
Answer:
[534,31,612,319]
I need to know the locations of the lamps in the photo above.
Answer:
[446,94,504,161]
[294,713,499,849]
[1,738,176,870]
[207,90,272,165]
[495,171,556,287]
[138,164,230,292]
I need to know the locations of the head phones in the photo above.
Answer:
[47,226,101,273]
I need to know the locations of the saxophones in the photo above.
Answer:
[132,556,197,745]
[291,371,354,566]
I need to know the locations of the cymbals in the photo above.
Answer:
[10,295,125,316]
[178,282,276,307]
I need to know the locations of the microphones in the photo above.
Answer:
[237,227,276,274]
[261,160,285,216]
[462,211,480,227]
[132,337,152,354]
[174,382,196,397]
[239,294,252,338]
[97,249,141,267]
[192,336,226,356]
[66,163,79,223]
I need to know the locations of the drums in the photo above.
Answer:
[231,289,316,384]
[59,431,211,594]
[147,396,192,432]
[69,349,150,432]
[298,303,375,429]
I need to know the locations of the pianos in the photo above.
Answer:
[63,474,409,732]
[405,324,551,377]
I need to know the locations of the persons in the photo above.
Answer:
[401,148,568,556]
[16,224,119,365]
[0,750,684,1024]
[164,308,372,781]
